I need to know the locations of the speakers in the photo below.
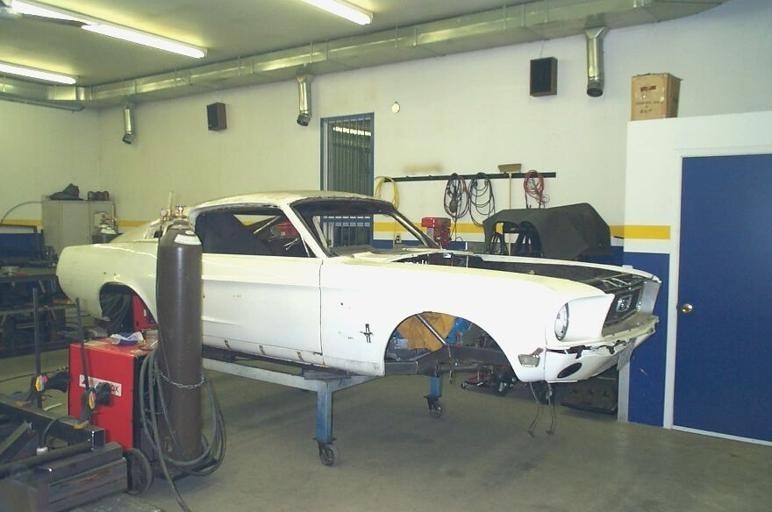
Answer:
[529,57,557,97]
[206,103,226,132]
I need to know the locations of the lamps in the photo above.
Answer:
[302,0,375,25]
[0,60,76,88]
[1,0,207,61]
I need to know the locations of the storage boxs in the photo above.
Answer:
[630,74,681,120]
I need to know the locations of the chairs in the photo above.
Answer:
[204,222,270,254]
